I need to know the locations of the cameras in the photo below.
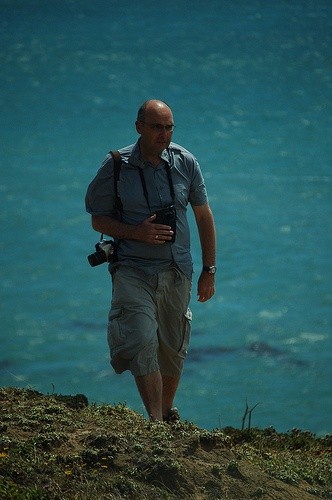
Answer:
[149,208,176,242]
[88,239,119,267]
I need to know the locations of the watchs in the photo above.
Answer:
[203,266,216,274]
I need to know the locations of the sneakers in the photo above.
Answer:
[163,407,180,423]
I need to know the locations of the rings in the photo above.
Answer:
[156,235,159,239]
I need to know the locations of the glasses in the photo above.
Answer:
[139,119,175,132]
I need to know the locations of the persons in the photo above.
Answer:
[85,100,216,419]
